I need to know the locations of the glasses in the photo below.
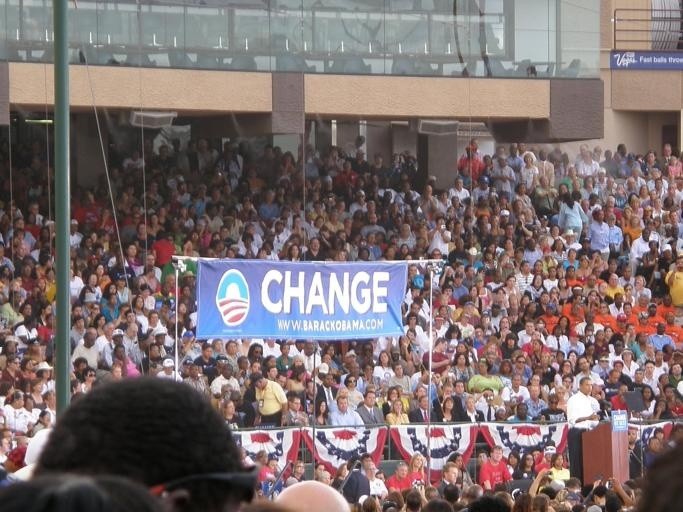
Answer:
[151,464,259,504]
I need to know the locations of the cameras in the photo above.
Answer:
[605,480,612,490]
[486,395,493,401]
[440,224,446,230]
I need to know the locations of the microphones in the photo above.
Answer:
[598,399,612,408]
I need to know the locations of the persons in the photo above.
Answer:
[0,135,683,512]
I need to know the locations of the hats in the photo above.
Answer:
[5,144,671,388]
